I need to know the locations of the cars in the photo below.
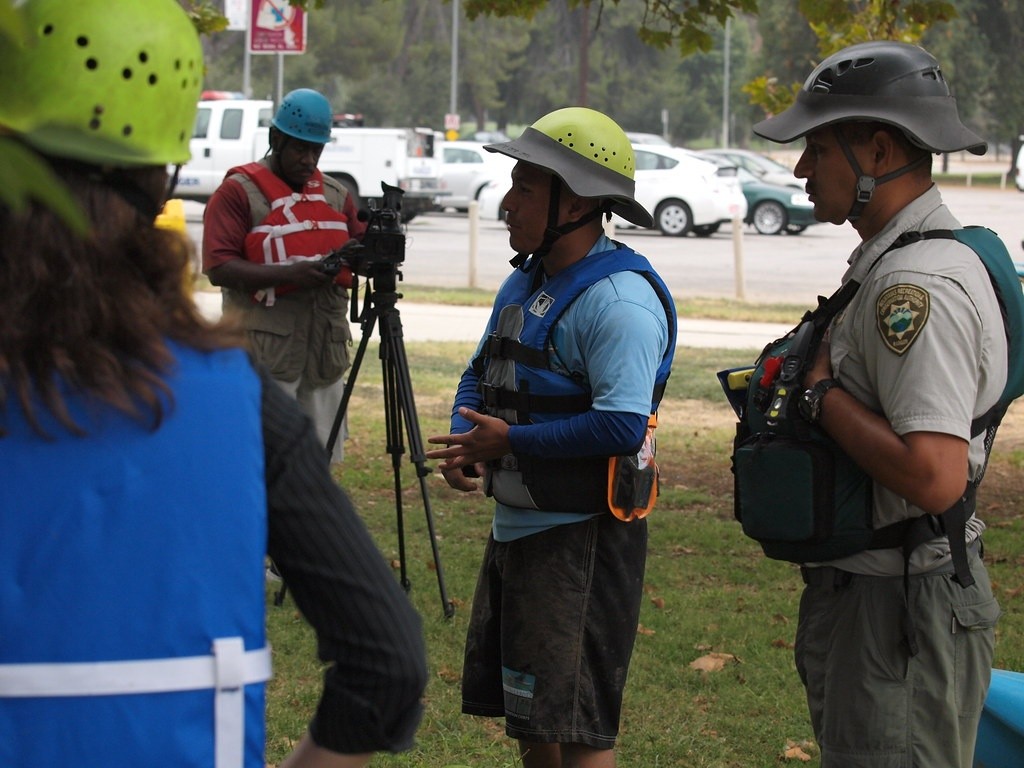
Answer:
[685,148,825,236]
[435,139,496,213]
[477,131,748,237]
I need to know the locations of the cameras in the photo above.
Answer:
[337,181,406,264]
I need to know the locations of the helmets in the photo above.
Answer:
[750,41,988,156]
[483,107,654,231]
[271,88,334,144]
[1,0,204,168]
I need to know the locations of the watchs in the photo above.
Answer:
[797,378,848,425]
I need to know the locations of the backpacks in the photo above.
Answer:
[729,224,1024,587]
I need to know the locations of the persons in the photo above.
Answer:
[751,41,1024,767]
[2,0,430,768]
[202,89,368,581]
[424,106,678,767]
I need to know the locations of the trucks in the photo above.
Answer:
[160,88,444,229]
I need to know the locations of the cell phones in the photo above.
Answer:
[318,264,341,277]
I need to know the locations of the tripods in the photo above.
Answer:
[272,264,458,617]
